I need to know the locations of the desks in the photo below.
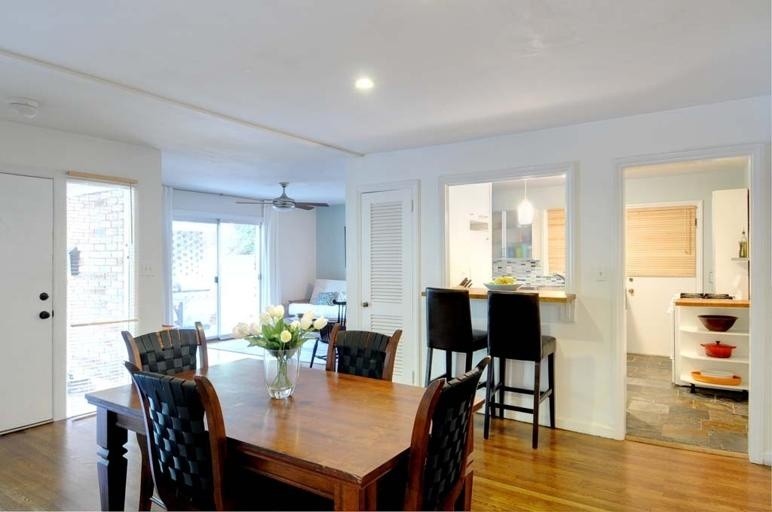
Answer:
[83,357,435,510]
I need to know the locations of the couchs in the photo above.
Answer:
[288,279,346,321]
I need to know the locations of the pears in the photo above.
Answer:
[495,276,514,284]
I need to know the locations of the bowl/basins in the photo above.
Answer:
[697,315,739,332]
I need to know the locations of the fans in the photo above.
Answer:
[235,182,329,211]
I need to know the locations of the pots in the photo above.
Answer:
[699,340,737,358]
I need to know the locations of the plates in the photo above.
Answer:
[482,280,524,292]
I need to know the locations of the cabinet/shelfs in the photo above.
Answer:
[672,307,749,393]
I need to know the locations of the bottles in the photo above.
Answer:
[739,229,748,258]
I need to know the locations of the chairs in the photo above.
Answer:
[123,359,290,512]
[326,321,403,382]
[122,320,209,510]
[402,354,494,512]
[483,289,556,450]
[422,287,497,420]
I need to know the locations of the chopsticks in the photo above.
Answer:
[459,277,473,288]
